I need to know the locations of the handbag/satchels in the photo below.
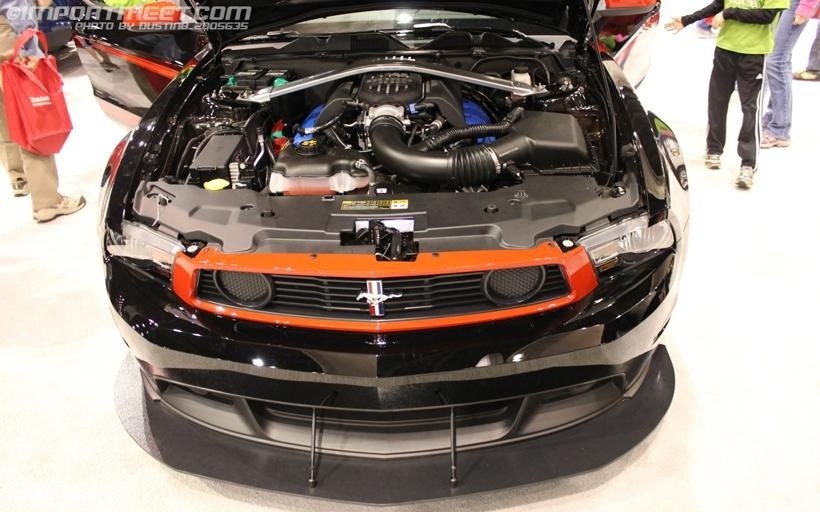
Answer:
[0,55,74,156]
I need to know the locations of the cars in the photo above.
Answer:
[70,0,688,504]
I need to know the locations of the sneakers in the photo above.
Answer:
[9,179,30,197]
[706,150,720,167]
[32,196,86,222]
[736,163,758,189]
[793,70,820,80]
[760,133,790,148]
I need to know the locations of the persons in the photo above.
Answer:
[661,0,793,190]
[693,1,820,149]
[0,1,87,224]
